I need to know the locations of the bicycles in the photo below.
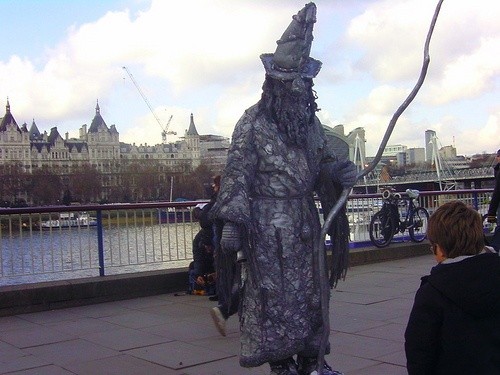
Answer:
[368,188,431,248]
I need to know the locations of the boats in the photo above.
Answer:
[36,211,97,228]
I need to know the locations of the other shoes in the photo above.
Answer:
[212,306,227,336]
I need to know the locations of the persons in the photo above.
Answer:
[405,200,500,374]
[186,175,237,337]
[487,149,500,249]
[207,2,356,375]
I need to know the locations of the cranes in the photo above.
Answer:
[121,65,178,144]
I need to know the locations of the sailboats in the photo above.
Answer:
[313,132,459,242]
[155,172,210,223]
